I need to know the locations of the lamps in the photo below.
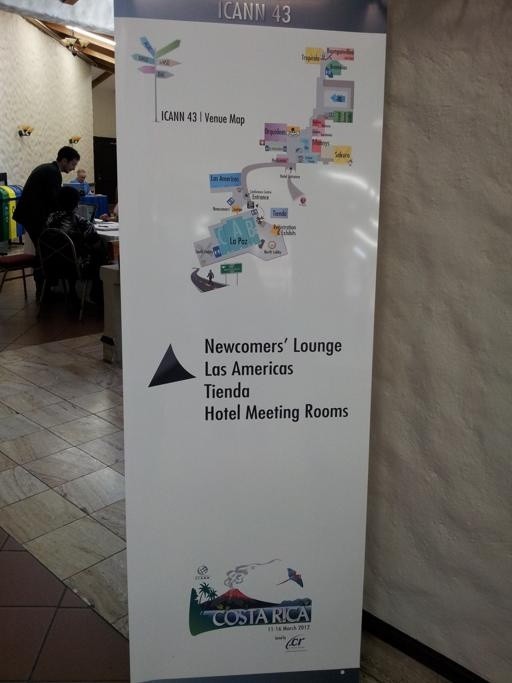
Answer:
[68,134,81,144]
[19,123,33,136]
[59,29,91,55]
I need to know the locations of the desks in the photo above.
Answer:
[87,216,119,262]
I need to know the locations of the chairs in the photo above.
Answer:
[1,197,102,319]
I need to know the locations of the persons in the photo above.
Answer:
[70,169,91,195]
[11,145,80,305]
[46,187,108,306]
[99,202,118,221]
